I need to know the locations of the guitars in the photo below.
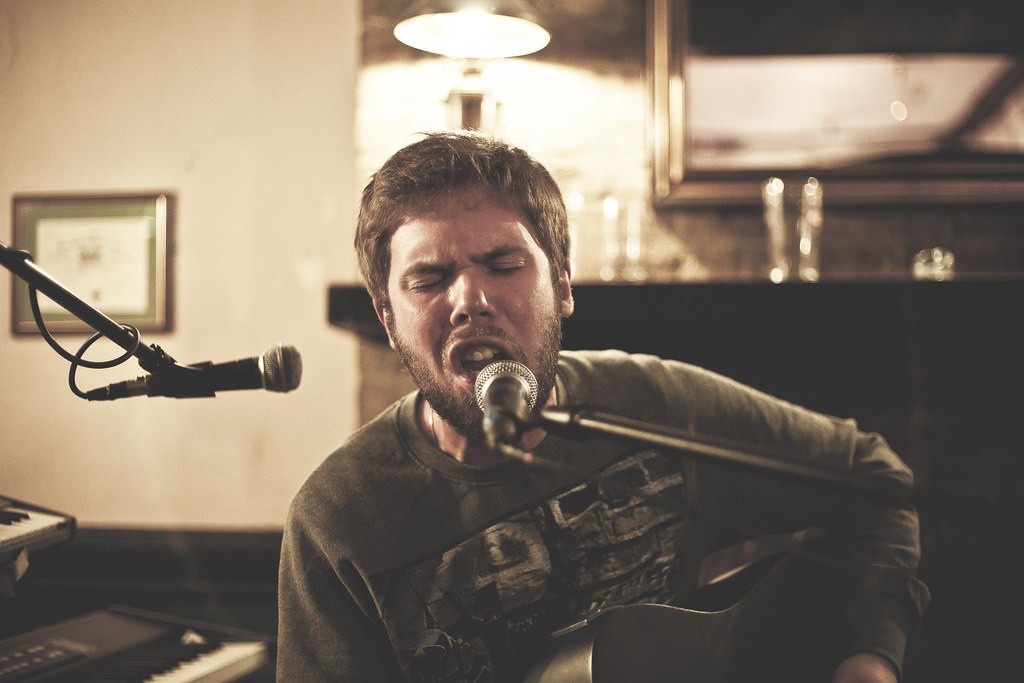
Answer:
[520,522,850,683]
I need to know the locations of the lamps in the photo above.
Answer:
[391,1,552,131]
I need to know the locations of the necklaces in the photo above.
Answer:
[429,406,442,451]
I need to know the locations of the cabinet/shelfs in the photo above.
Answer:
[327,280,1023,683]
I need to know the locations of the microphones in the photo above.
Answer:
[88,344,302,402]
[474,359,539,448]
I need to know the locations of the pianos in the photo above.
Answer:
[0,494,78,564]
[0,599,277,683]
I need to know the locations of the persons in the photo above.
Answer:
[273,133,919,683]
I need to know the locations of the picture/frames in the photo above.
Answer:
[643,0,1023,209]
[7,192,170,334]
